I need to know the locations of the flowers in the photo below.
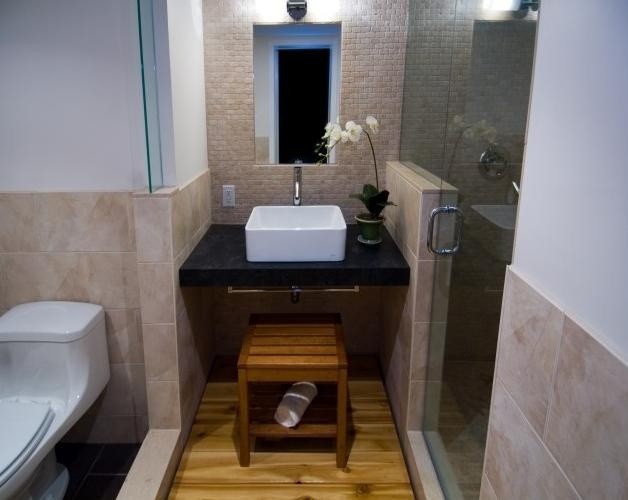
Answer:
[312,114,401,218]
[427,102,507,182]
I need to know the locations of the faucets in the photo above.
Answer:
[295,158,303,164]
[505,180,519,204]
[293,167,302,206]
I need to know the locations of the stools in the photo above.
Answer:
[235,310,353,467]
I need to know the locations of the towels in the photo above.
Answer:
[274,381,318,430]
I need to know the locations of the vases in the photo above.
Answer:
[354,211,386,245]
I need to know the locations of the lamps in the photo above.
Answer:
[478,1,538,20]
[283,0,309,23]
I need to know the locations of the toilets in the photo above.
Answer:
[1,300,111,500]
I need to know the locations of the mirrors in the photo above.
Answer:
[248,20,345,171]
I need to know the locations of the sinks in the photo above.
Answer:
[469,204,518,262]
[244,205,348,263]
